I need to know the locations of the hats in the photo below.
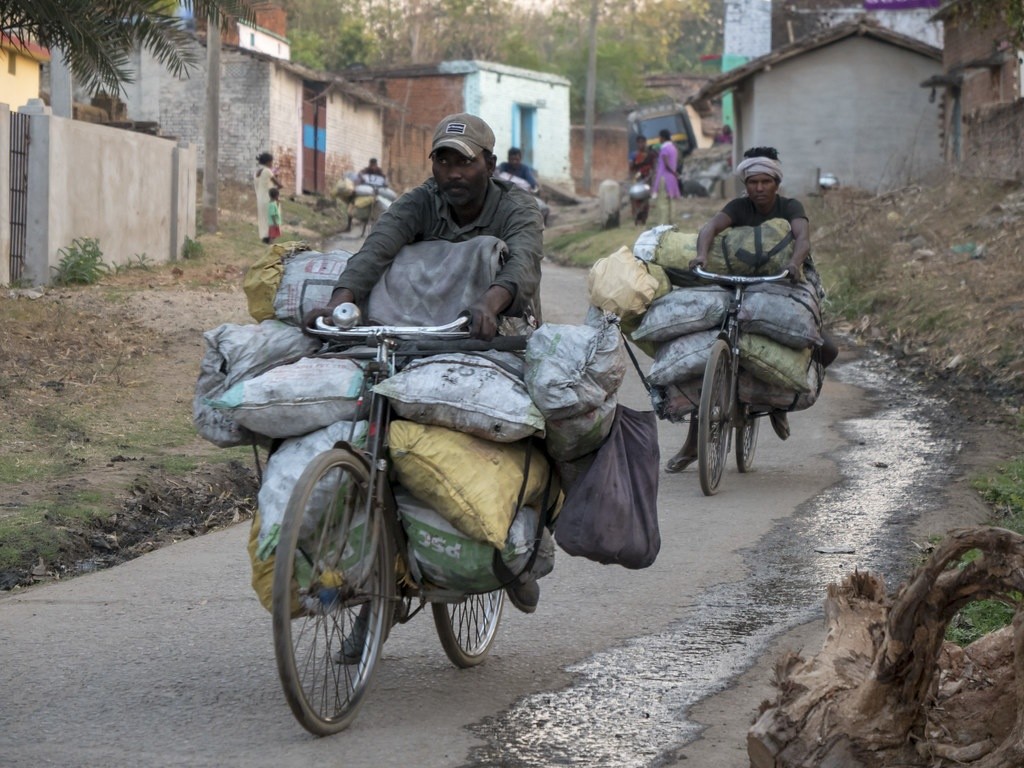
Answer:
[428,113,495,160]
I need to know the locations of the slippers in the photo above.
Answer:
[770,411,790,440]
[665,455,699,473]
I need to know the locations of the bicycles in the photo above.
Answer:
[270,300,557,738]
[688,258,793,499]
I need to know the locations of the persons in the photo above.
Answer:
[302,112,544,664]
[628,123,733,225]
[254,153,284,244]
[665,146,811,472]
[343,159,388,232]
[494,148,541,197]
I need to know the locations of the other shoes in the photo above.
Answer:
[506,580,540,613]
[331,601,407,664]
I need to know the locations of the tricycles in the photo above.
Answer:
[348,182,387,238]
